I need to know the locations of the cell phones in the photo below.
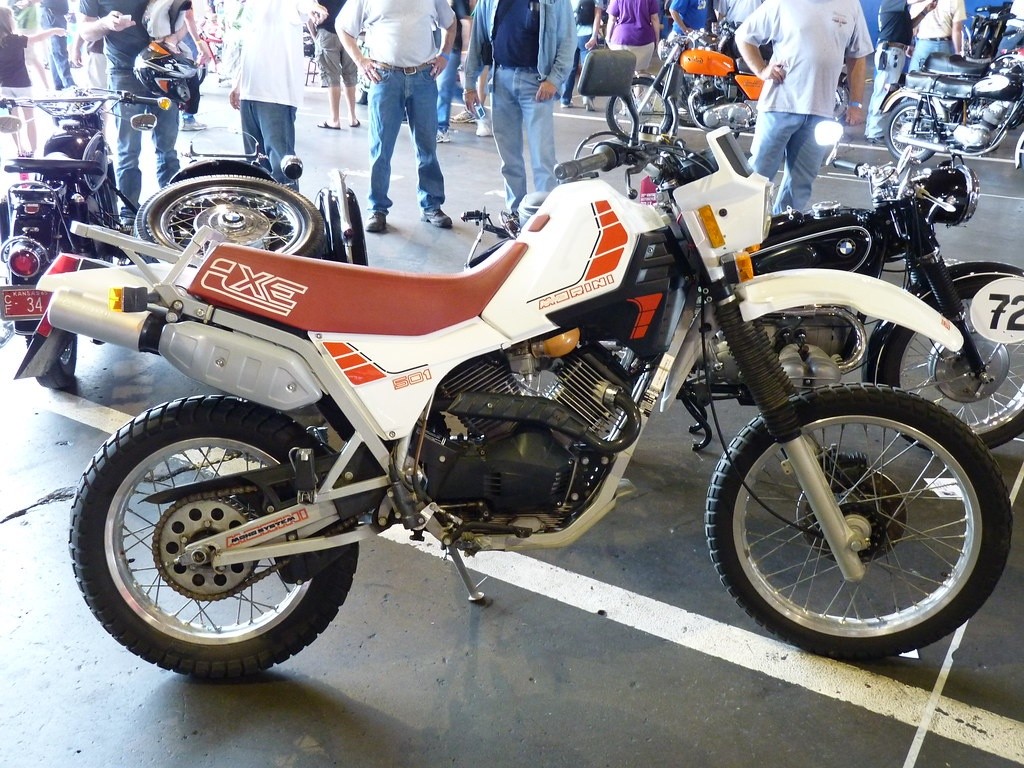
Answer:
[119,14,132,22]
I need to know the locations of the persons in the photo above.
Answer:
[910,0,967,88]
[734,0,874,215]
[865,0,937,145]
[0,0,739,234]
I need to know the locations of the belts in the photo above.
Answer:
[374,59,433,76]
[887,41,907,49]
[928,37,951,41]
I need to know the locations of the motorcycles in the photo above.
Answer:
[2,48,1014,684]
[607,21,853,145]
[1,83,369,390]
[463,147,1024,458]
[880,0,1024,172]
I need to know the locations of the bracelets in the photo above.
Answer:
[848,101,863,108]
[921,9,927,16]
[196,40,201,45]
[439,51,449,61]
[592,34,598,38]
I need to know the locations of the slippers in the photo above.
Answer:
[348,119,360,127]
[317,121,340,129]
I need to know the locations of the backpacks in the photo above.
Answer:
[575,0,596,25]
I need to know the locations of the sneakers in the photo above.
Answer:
[435,129,451,143]
[449,109,477,123]
[178,122,207,131]
[475,116,491,137]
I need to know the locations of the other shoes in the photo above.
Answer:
[585,105,595,111]
[365,211,386,232]
[560,101,574,108]
[120,215,134,233]
[420,206,452,228]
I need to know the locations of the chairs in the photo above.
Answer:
[304,26,319,86]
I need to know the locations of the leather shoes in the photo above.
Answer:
[867,136,887,146]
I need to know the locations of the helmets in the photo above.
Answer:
[133,41,199,103]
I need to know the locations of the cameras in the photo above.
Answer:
[472,104,485,120]
